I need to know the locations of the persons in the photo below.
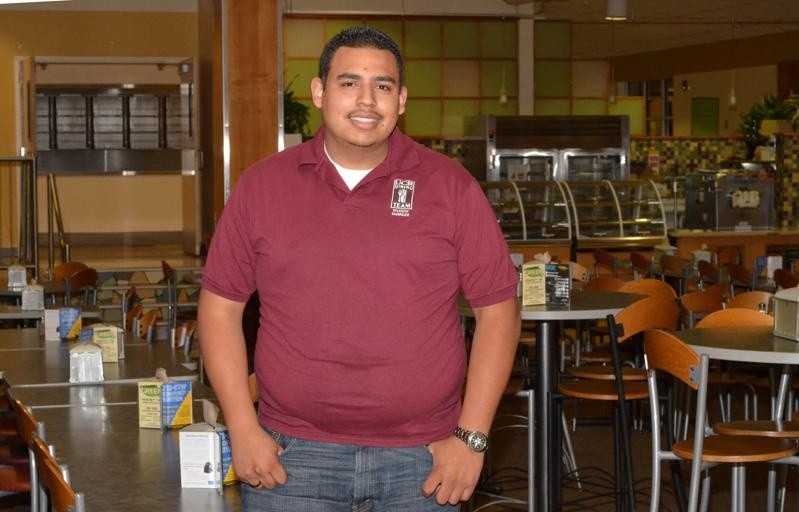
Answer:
[195,26,522,512]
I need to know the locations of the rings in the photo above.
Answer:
[251,482,261,488]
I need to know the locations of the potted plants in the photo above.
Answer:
[282,72,314,151]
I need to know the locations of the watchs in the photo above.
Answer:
[453,426,489,452]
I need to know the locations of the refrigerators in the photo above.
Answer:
[462,113,632,236]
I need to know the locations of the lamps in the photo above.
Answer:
[497,11,510,107]
[726,21,738,111]
[605,23,617,103]
[602,1,630,22]
